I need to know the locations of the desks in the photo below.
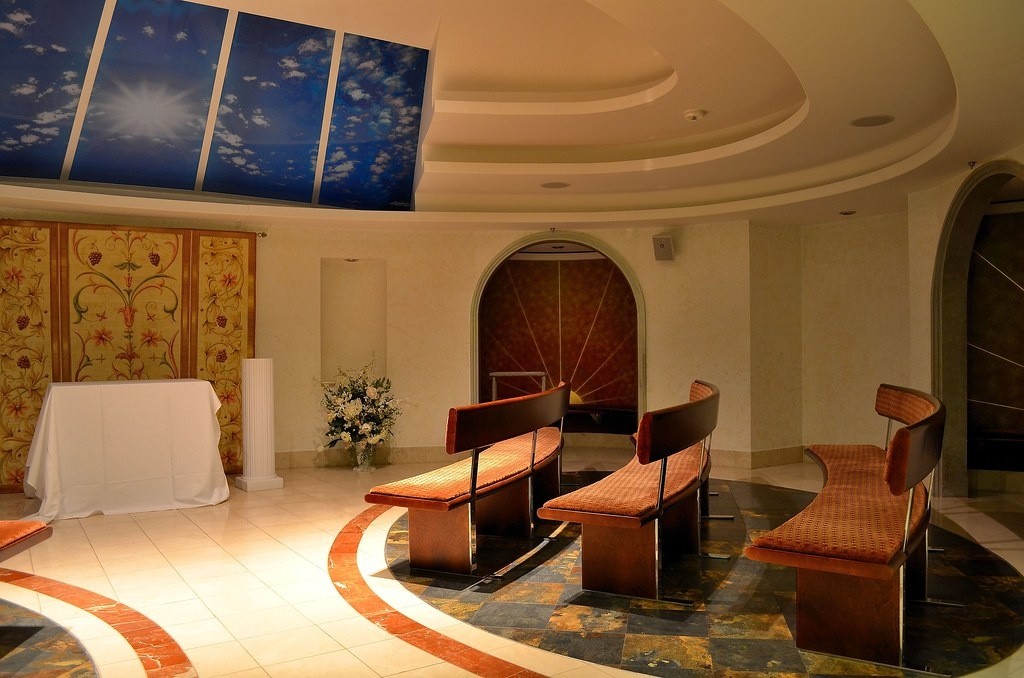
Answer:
[48,378,216,511]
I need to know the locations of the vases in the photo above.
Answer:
[352,440,380,472]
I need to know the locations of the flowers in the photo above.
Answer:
[312,369,420,452]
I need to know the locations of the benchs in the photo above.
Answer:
[744,383,948,668]
[536,380,720,602]
[364,378,573,577]
